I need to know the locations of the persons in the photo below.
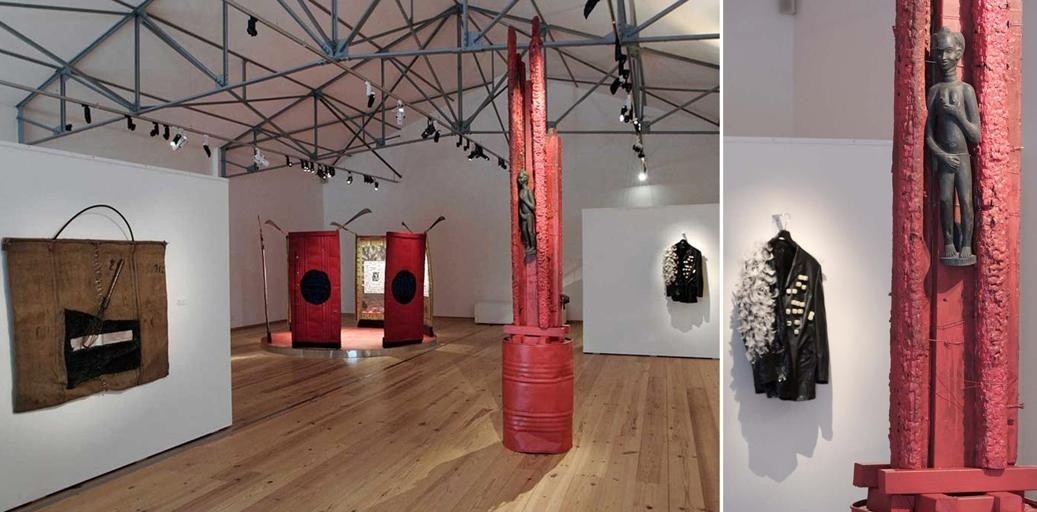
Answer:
[923,25,980,267]
[517,169,537,255]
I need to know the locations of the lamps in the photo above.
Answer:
[395,97,406,127]
[125,114,212,159]
[420,119,507,170]
[610,44,649,181]
[284,155,379,191]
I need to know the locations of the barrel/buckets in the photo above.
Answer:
[500,335,574,458]
